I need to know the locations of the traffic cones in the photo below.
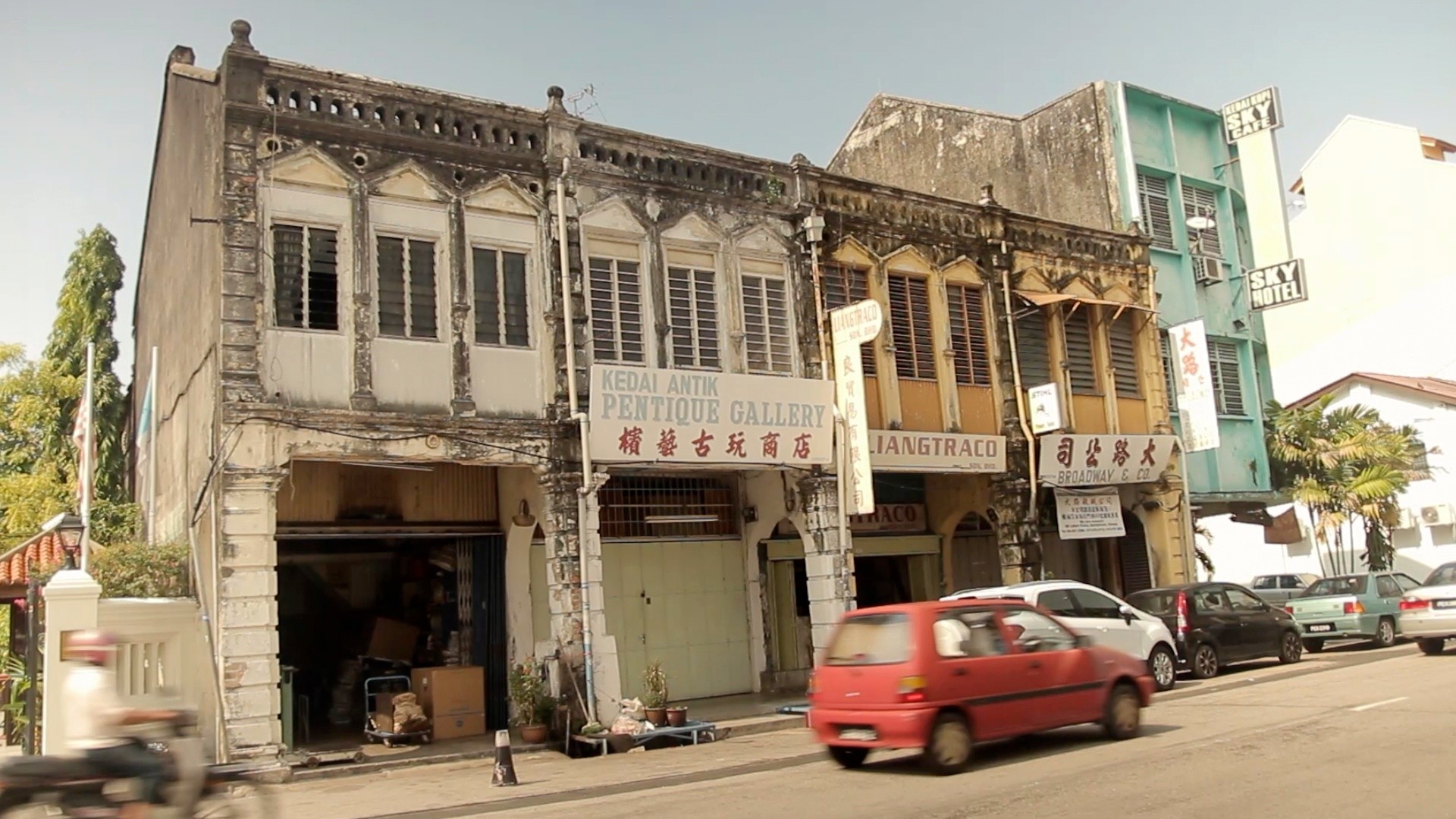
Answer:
[492,730,519,787]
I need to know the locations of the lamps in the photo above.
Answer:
[51,512,86,571]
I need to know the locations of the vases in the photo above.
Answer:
[666,705,689,728]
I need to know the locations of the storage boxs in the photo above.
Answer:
[375,691,400,733]
[409,663,487,741]
[355,613,421,667]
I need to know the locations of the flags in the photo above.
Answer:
[136,374,150,514]
[71,387,96,506]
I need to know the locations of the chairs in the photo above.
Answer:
[966,627,1001,659]
[1201,595,1220,611]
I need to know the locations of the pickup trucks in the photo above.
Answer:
[1200,572,1323,615]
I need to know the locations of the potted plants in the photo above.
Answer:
[641,658,668,727]
[509,659,558,745]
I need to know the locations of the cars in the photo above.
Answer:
[1127,584,1304,678]
[809,601,1151,773]
[1284,572,1422,652]
[1397,562,1456,656]
[938,580,1180,696]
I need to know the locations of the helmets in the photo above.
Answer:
[63,628,117,665]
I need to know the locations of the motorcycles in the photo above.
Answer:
[2,704,286,819]
